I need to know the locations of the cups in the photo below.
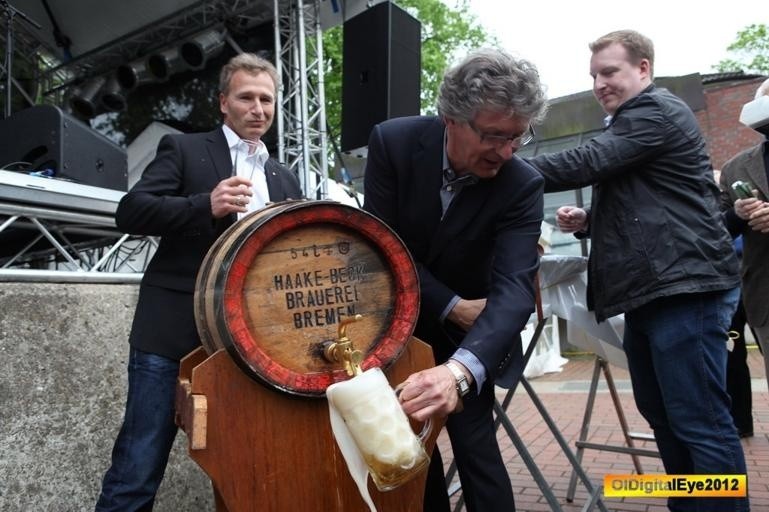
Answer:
[330,366,437,491]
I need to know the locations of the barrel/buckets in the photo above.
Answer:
[192,199,422,398]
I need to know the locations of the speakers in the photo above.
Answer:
[1,103,128,192]
[340,1,422,151]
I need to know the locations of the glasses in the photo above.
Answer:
[469,120,535,149]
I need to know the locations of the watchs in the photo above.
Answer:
[444,361,476,417]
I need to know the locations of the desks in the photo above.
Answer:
[443,255,608,511]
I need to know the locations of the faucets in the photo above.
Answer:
[323,314,364,381]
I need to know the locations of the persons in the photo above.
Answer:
[712,167,754,442]
[524,29,752,511]
[718,74,769,386]
[362,44,546,512]
[94,51,304,512]
[236,193,245,207]
